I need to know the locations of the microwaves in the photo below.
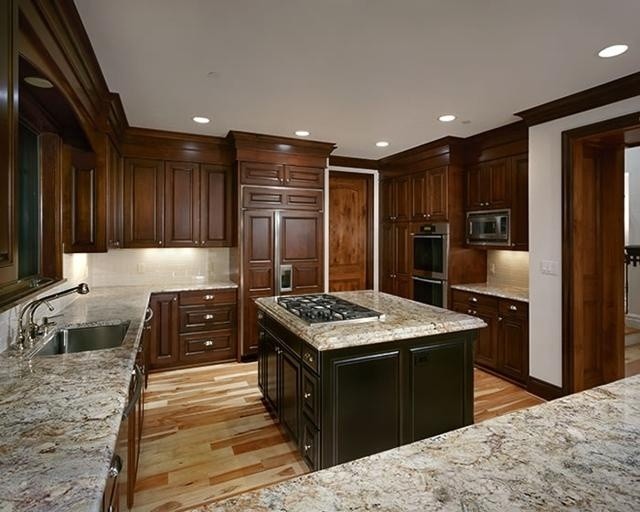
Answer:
[466,214,508,241]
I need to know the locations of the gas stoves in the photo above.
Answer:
[277,292,385,328]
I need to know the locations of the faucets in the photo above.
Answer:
[16,283,90,349]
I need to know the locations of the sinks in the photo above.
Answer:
[23,320,130,360]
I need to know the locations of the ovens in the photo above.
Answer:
[409,222,447,309]
